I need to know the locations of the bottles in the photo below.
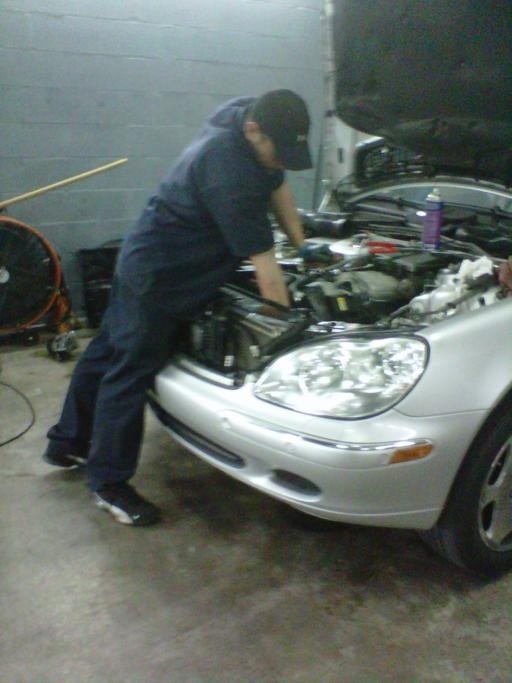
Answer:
[422,189,442,252]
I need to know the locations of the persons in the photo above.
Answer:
[43,89,314,526]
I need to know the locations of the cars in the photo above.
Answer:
[142,0,512,581]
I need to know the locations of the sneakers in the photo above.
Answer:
[88,479,164,527]
[43,434,93,468]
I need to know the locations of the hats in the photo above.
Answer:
[253,89,313,171]
